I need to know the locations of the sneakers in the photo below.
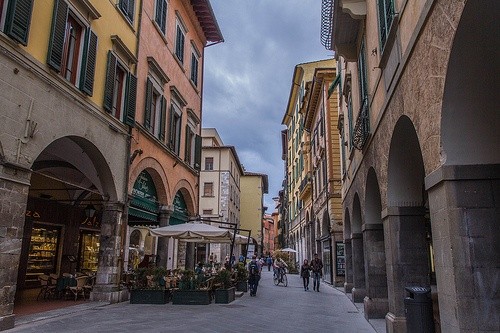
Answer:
[250,289,255,296]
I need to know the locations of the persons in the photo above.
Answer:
[301,259,313,291]
[250,254,289,283]
[309,253,324,292]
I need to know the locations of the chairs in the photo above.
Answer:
[36,271,96,303]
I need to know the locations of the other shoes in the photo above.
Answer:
[303,286,321,293]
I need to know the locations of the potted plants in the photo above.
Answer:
[172,270,211,305]
[232,261,249,292]
[129,266,170,305]
[214,270,236,304]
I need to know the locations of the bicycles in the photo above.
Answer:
[273,265,289,287]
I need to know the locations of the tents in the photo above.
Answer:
[149,222,256,266]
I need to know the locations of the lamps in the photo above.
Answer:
[79,202,97,220]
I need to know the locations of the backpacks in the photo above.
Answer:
[250,260,258,279]
